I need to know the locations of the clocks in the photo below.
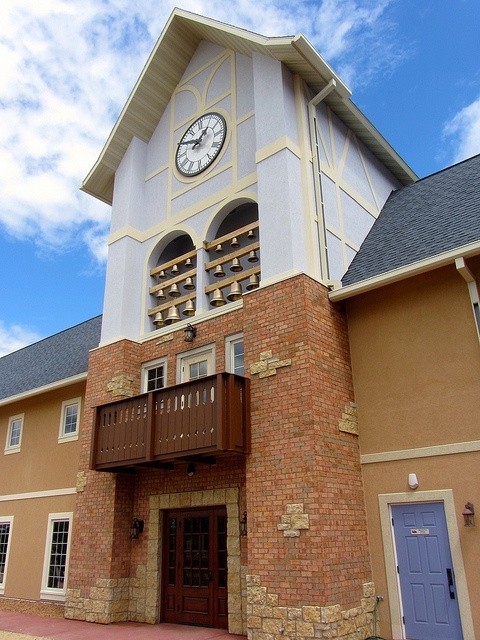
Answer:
[174,111,228,180]
